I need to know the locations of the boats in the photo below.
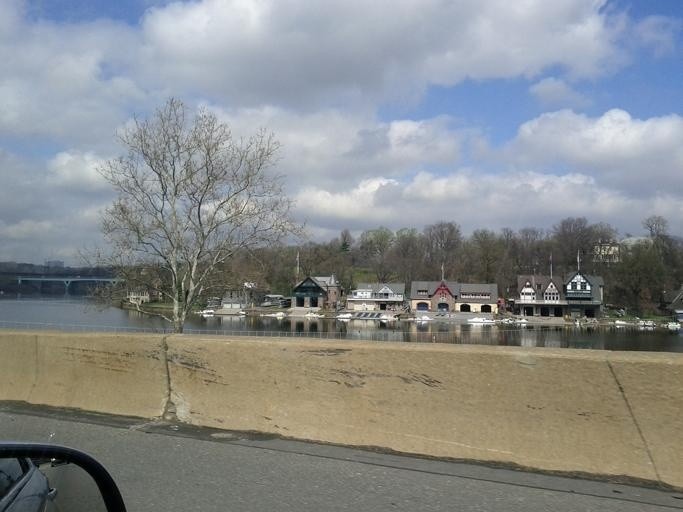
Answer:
[574,315,599,325]
[304,311,327,319]
[237,310,248,315]
[336,312,353,319]
[607,316,681,331]
[467,316,529,325]
[201,309,216,314]
[267,311,289,318]
[381,313,434,322]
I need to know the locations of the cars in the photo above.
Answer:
[1,458,62,511]
